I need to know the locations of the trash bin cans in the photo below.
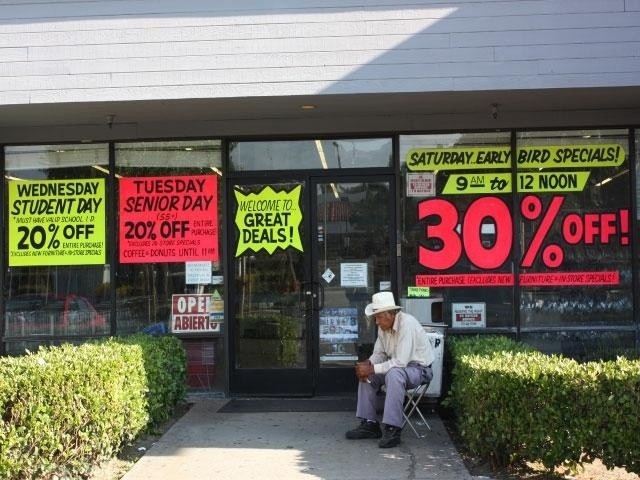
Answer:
[400,298,448,398]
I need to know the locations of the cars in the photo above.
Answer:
[93,286,151,311]
[5,291,106,338]
[279,304,305,320]
[521,289,633,318]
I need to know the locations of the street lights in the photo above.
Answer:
[247,255,256,315]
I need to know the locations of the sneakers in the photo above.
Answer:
[346,419,382,438]
[380,425,401,447]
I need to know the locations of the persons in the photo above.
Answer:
[346,291,436,448]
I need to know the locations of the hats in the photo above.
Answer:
[365,292,404,316]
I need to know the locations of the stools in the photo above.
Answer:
[381,382,433,440]
